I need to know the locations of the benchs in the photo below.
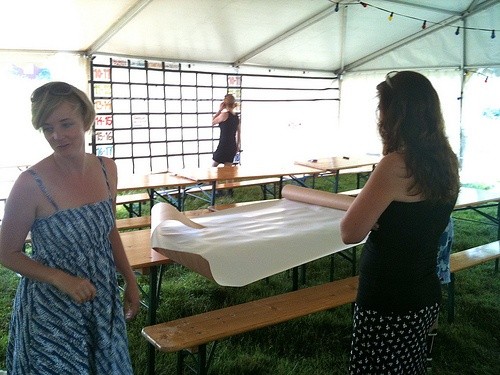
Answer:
[141,240,500,374]
[117,163,377,218]
[115,199,289,231]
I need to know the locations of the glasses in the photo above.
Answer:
[30,83,80,102]
[385,71,399,89]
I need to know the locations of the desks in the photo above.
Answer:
[118,187,500,375]
[0,155,385,216]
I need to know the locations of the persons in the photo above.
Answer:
[340,70,460,375]
[0,82,140,375]
[211,94,241,197]
